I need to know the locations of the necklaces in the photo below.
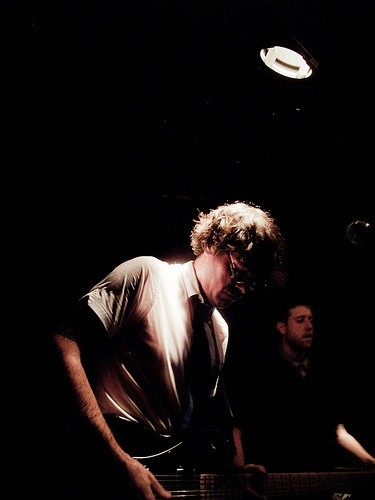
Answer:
[230,298,375,470]
[47,200,291,500]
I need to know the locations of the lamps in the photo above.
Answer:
[259,1,322,79]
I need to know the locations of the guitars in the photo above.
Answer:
[65,411,375,500]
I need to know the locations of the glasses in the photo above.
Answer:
[226,250,269,295]
